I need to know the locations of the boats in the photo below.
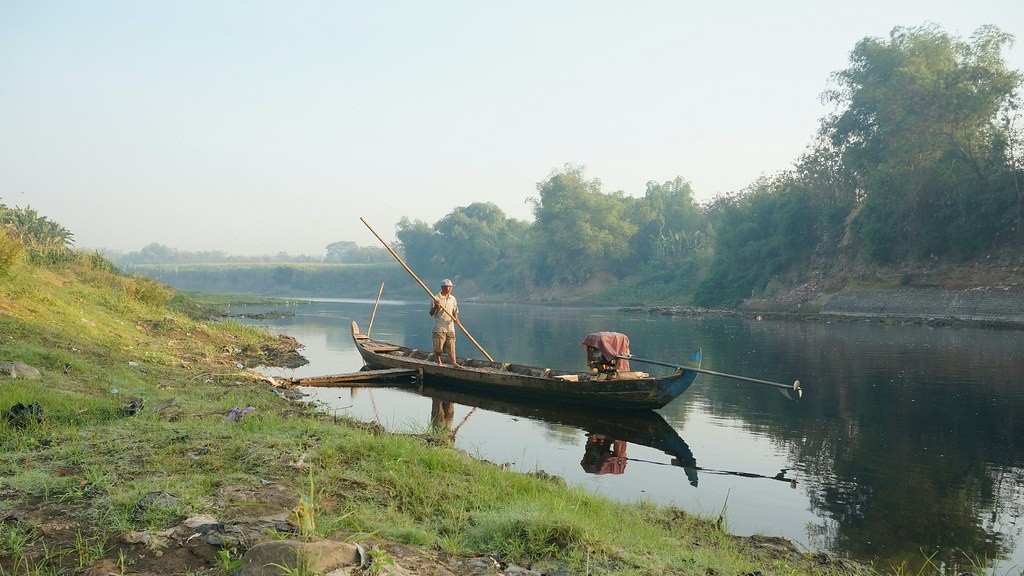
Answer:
[358,365,700,490]
[351,320,704,408]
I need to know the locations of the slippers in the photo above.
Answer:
[454,363,462,368]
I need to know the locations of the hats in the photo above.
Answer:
[441,279,453,287]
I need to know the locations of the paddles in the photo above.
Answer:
[360,217,495,363]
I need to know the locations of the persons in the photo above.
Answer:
[428,279,465,368]
[428,397,454,437]
